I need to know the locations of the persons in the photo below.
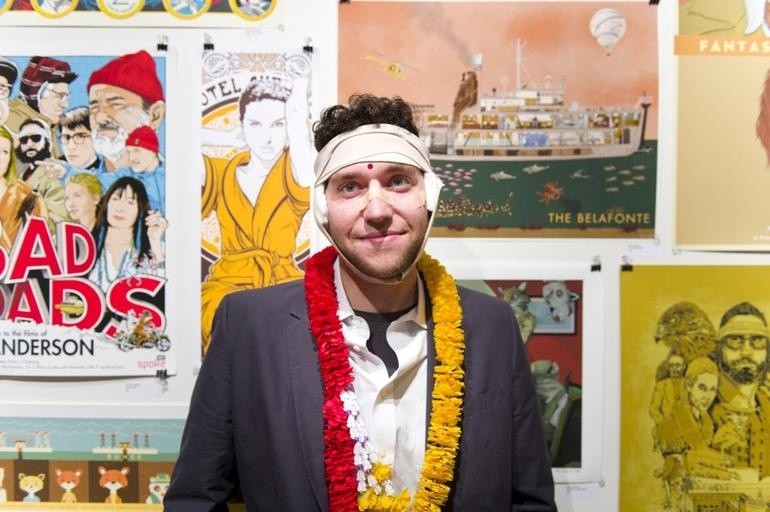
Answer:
[60,173,105,242]
[8,57,77,161]
[160,93,560,512]
[86,49,166,191]
[652,302,770,510]
[202,72,314,359]
[0,58,17,123]
[0,124,60,256]
[112,124,163,214]
[654,352,743,511]
[55,105,100,172]
[15,116,73,224]
[82,175,169,294]
[648,354,688,423]
